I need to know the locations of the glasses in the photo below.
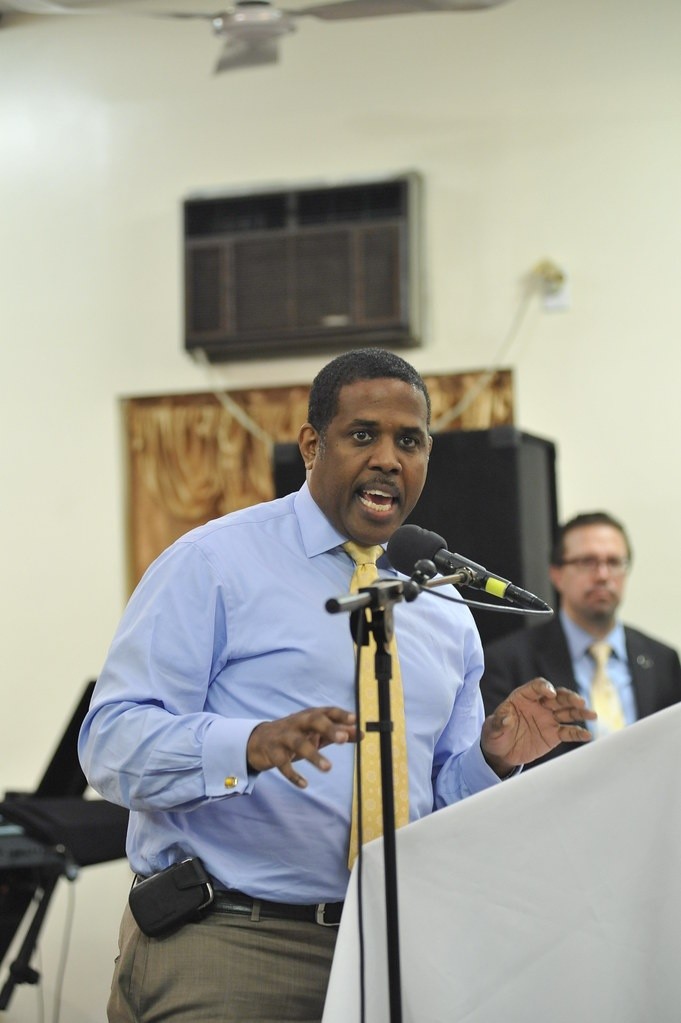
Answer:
[563,555,628,572]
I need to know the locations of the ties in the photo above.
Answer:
[340,541,409,872]
[588,641,624,739]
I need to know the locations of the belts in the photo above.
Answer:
[136,874,344,931]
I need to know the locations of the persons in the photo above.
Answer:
[480,511,681,774]
[78,348,597,1023]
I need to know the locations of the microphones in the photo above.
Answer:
[387,524,550,610]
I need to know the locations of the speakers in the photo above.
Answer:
[269,425,560,645]
